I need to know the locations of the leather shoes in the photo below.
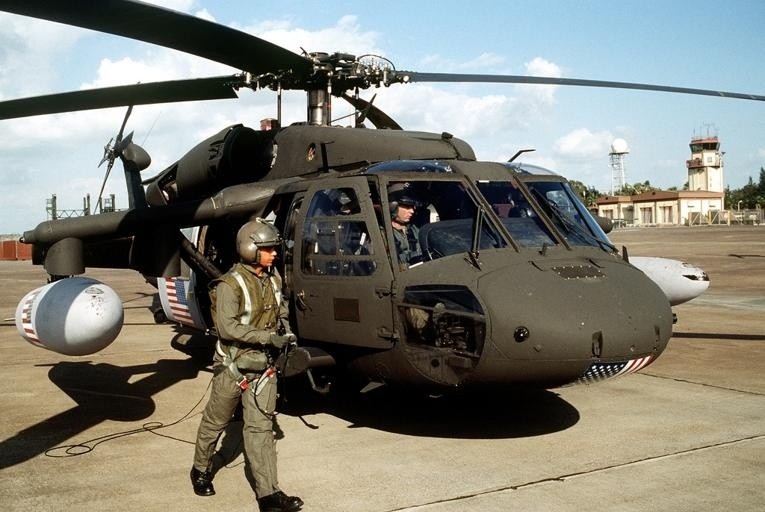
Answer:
[258,491,304,512]
[190,464,215,496]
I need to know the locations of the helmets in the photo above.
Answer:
[389,195,422,219]
[237,221,282,266]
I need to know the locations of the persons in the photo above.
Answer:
[189,221,304,511]
[360,192,428,274]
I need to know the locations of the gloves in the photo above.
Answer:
[267,333,298,352]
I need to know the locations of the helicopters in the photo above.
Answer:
[0,0,765,400]
